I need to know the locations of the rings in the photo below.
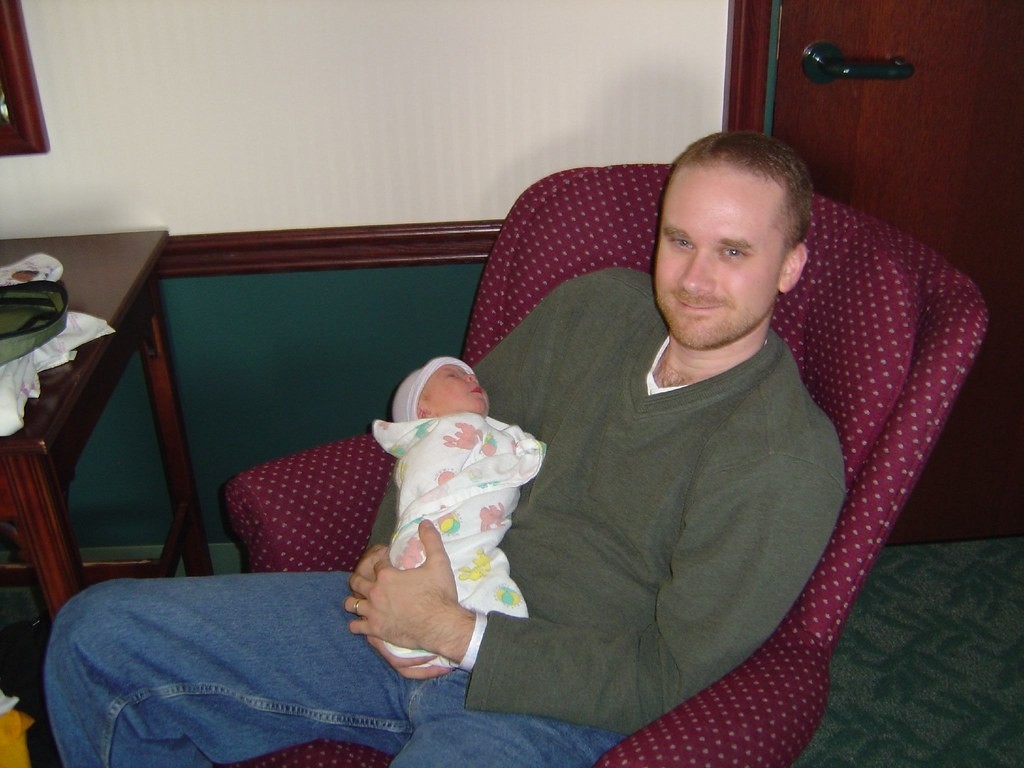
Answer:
[353,597,365,616]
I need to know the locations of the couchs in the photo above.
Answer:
[209,164,988,768]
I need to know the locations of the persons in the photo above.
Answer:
[390,356,544,670]
[44,127,852,768]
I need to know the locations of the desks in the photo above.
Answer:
[0,230,214,627]
